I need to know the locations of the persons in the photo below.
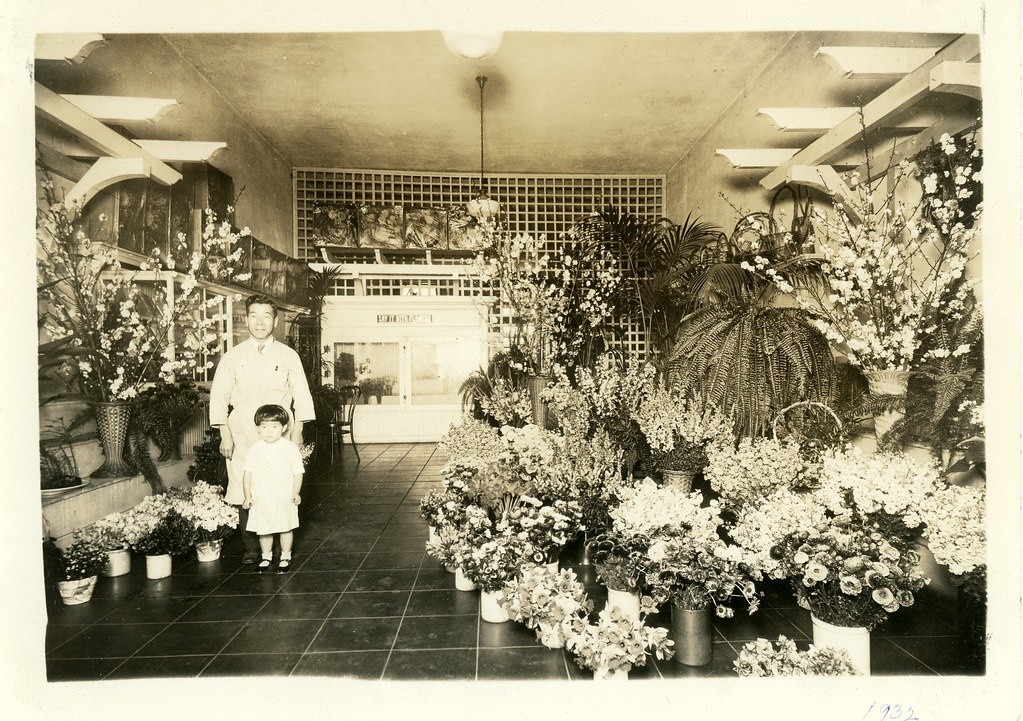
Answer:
[210,296,315,564]
[242,405,305,575]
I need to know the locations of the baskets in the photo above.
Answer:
[773,401,845,488]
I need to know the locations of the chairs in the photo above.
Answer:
[311,385,360,465]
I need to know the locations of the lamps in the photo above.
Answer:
[438,30,505,59]
[466,76,499,217]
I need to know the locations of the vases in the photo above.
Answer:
[592,664,629,681]
[59,574,96,605]
[146,553,173,579]
[100,546,132,578]
[196,538,223,562]
[605,587,641,622]
[940,448,977,486]
[669,601,713,666]
[454,567,477,591]
[481,588,511,622]
[429,525,440,542]
[447,565,457,574]
[811,612,870,675]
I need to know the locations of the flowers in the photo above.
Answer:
[35,142,315,582]
[421,118,985,675]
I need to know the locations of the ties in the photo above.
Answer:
[257,344,265,354]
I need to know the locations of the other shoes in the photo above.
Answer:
[242,549,261,563]
[276,559,291,574]
[253,559,274,574]
